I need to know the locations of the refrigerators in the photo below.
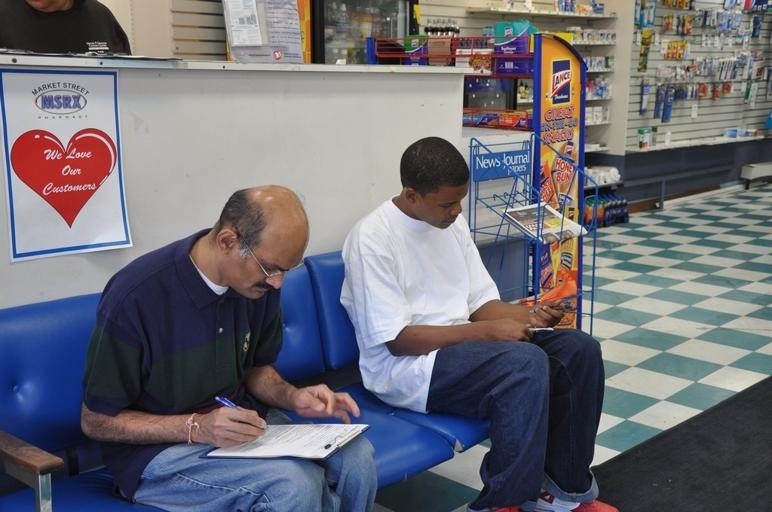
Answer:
[299,0,419,66]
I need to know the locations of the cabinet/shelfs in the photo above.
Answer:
[365,31,588,335]
[467,8,622,193]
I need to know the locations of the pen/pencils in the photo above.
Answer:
[215,396,271,433]
[528,305,564,313]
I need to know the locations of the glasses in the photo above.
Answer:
[236,231,306,280]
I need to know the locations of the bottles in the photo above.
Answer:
[585,194,629,228]
[323,2,395,65]
[638,129,649,148]
[424,18,460,37]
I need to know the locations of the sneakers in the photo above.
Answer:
[493,483,622,512]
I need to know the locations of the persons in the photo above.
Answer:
[0,0,134,55]
[337,133,620,512]
[73,182,381,512]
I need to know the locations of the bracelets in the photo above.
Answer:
[185,412,200,445]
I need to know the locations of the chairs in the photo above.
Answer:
[0,251,491,512]
